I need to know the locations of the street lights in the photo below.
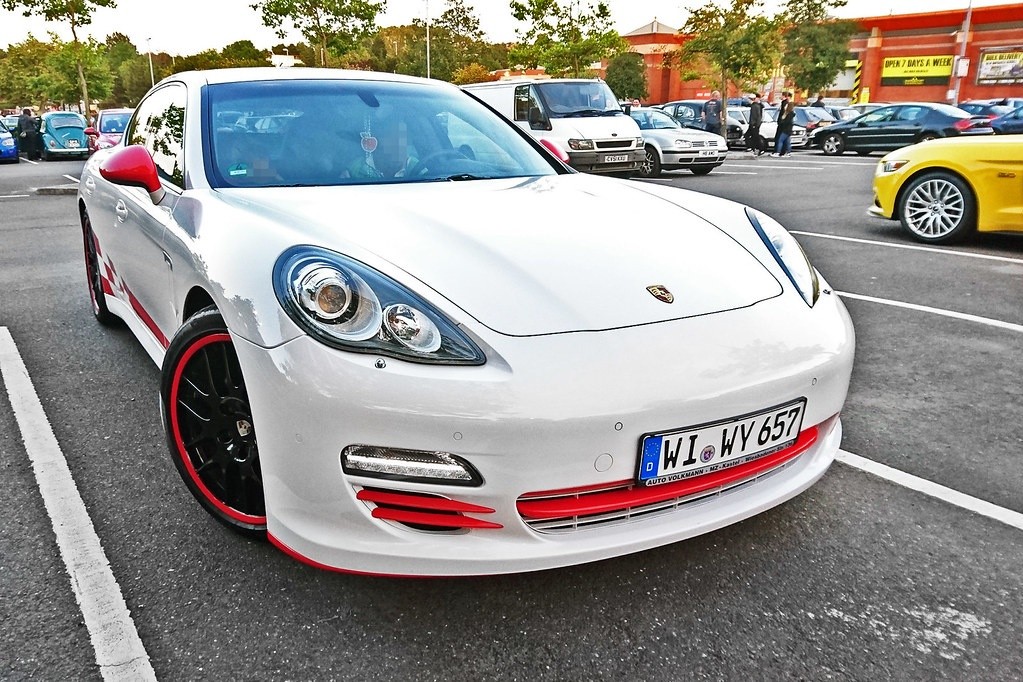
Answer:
[146,37,154,87]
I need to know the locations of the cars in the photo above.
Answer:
[810,101,986,153]
[82,108,137,155]
[0,122,19,163]
[990,106,1023,135]
[765,106,836,126]
[650,100,743,150]
[622,107,729,179]
[968,96,1023,117]
[995,154,996,157]
[867,132,1023,245]
[824,106,862,121]
[958,99,1016,116]
[40,112,90,161]
[848,102,894,120]
[726,107,809,156]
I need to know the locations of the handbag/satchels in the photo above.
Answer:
[20,131,27,138]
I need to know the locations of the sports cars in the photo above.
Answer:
[77,65,857,576]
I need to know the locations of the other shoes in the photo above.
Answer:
[782,153,791,157]
[770,153,780,157]
[758,149,766,156]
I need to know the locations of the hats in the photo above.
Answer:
[748,95,756,99]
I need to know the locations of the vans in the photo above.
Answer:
[459,77,646,181]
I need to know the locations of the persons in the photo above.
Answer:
[811,95,825,109]
[703,91,723,135]
[339,120,428,179]
[770,91,794,158]
[744,94,765,156]
[633,98,642,107]
[18,109,41,161]
[752,93,765,153]
